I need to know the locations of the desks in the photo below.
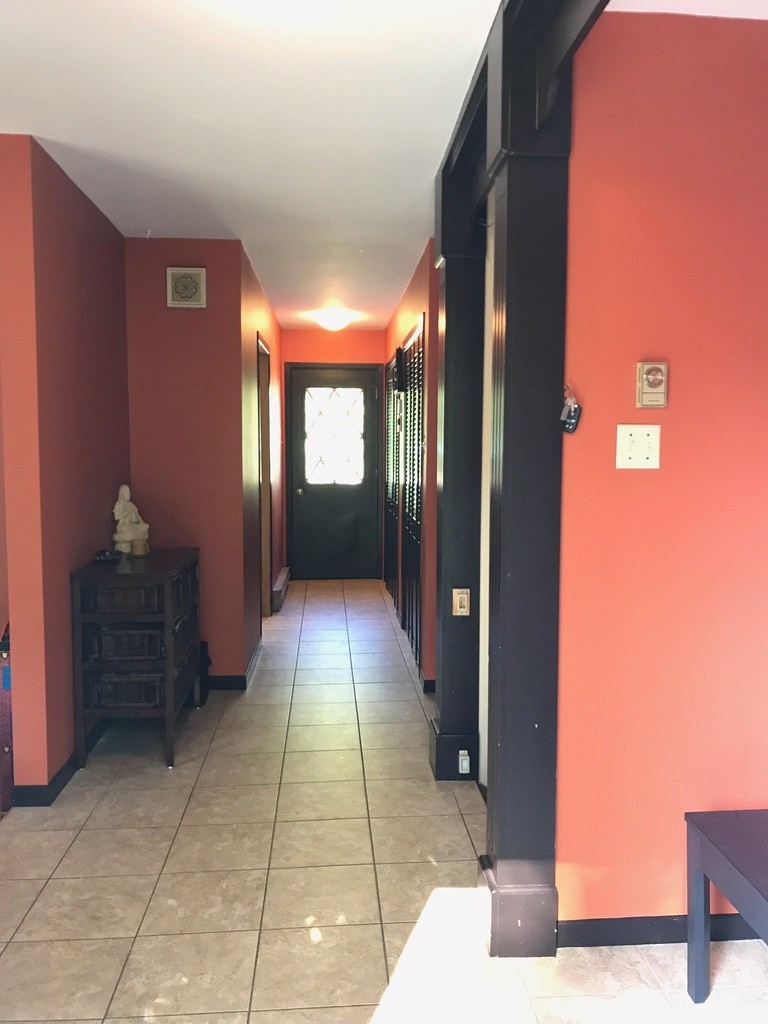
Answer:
[69,546,202,770]
[684,808,768,1003]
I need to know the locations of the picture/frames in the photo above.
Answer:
[167,266,207,308]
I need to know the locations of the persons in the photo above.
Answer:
[112,484,150,535]
